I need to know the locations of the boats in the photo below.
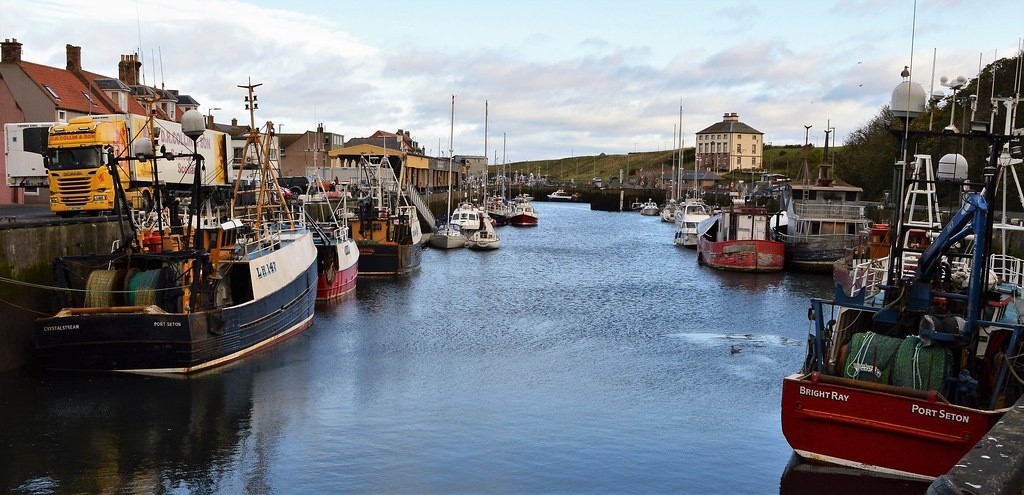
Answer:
[637,0,1023,483]
[0,13,539,382]
[547,189,572,202]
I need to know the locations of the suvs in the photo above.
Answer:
[268,175,317,197]
[233,177,261,188]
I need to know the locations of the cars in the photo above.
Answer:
[315,177,334,192]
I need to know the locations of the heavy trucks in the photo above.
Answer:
[42,110,235,217]
